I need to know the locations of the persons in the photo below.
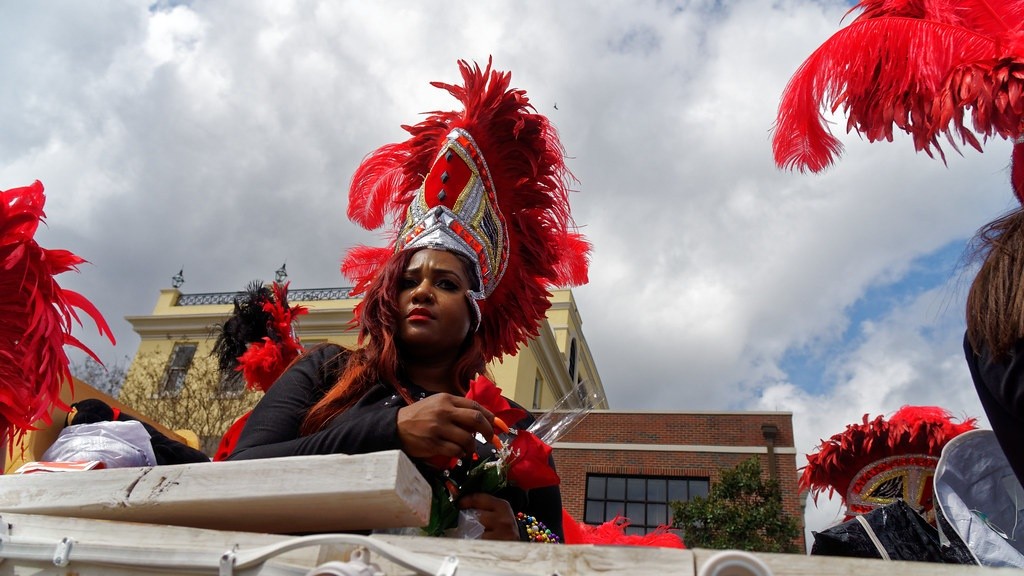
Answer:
[963,207,1024,491]
[225,243,564,545]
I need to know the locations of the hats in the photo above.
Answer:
[214,284,306,397]
[796,407,981,533]
[341,55,588,365]
[65,398,114,428]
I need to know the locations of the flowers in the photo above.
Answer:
[373,374,561,539]
